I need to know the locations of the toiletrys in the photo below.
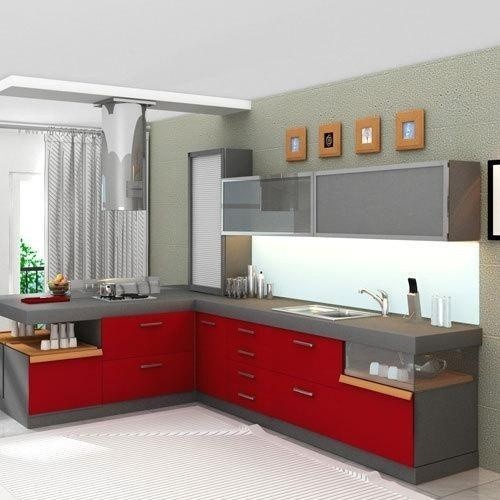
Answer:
[442,295,452,328]
[247,264,257,299]
[431,294,440,326]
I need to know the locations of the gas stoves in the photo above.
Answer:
[91,293,159,304]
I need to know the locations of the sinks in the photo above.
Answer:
[271,303,383,322]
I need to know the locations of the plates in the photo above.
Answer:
[48,291,67,297]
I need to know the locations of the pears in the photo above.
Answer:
[48,272,68,291]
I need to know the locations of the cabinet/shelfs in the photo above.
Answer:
[273,328,343,444]
[340,377,480,485]
[228,320,272,417]
[29,351,102,415]
[195,312,228,402]
[102,311,193,400]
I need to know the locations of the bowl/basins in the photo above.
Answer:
[45,280,70,294]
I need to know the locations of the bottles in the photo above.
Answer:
[225,265,275,301]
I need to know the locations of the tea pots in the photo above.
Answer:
[398,352,448,380]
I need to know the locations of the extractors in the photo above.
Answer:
[93,97,158,214]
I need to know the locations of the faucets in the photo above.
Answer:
[359,288,391,317]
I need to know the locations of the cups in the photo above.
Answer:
[70,276,163,297]
[368,361,410,383]
[9,319,77,352]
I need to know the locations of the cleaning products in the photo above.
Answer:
[256,271,265,299]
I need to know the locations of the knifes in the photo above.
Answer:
[407,277,421,294]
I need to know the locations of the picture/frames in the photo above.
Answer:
[285,127,306,161]
[319,124,342,158]
[396,109,425,151]
[355,117,381,154]
[488,159,500,239]
